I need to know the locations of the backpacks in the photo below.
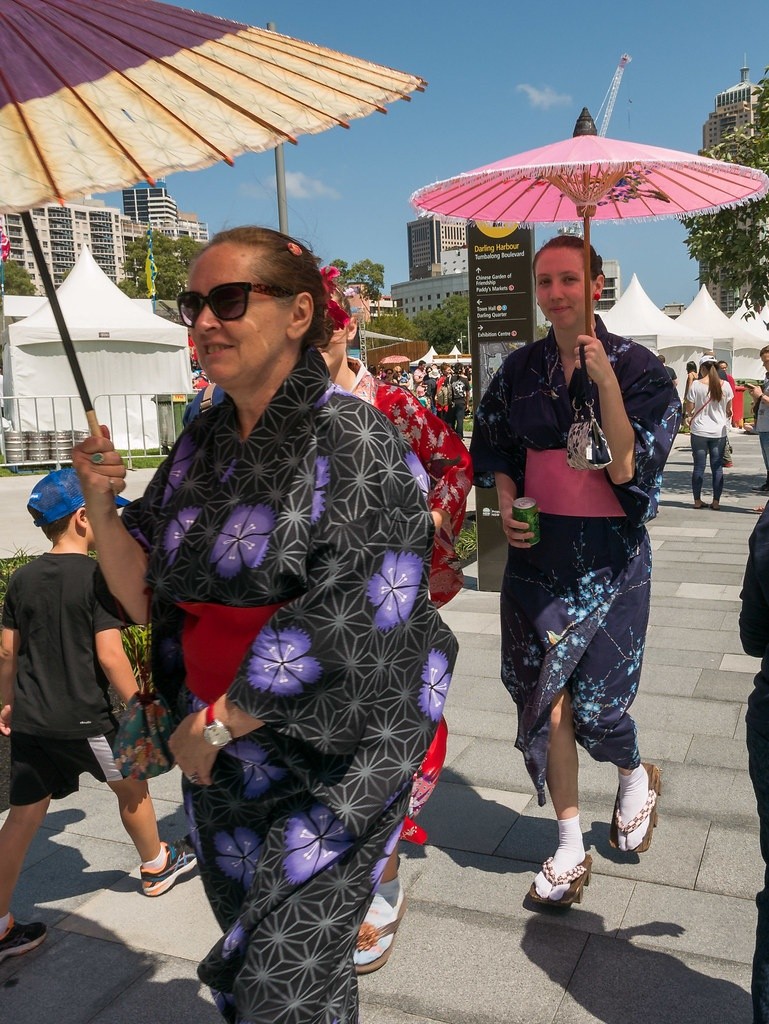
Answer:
[452,380,467,400]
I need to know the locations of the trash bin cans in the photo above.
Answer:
[151,394,198,455]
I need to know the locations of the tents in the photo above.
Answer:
[602,273,769,405]
[2,242,194,450]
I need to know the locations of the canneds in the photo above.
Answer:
[511,497,541,545]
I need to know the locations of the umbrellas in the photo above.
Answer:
[408,106,769,337]
[0,0,428,437]
[380,355,411,368]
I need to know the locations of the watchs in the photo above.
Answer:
[203,701,232,750]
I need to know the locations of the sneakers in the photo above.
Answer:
[138,832,201,897]
[0,915,49,962]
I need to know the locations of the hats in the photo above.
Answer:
[393,365,402,372]
[427,369,441,379]
[415,386,426,397]
[27,467,131,528]
[697,353,717,377]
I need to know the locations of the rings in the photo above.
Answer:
[91,453,104,464]
[109,477,114,488]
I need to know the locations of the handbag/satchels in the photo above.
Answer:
[563,347,615,471]
[433,384,450,406]
[723,429,736,470]
[109,584,179,783]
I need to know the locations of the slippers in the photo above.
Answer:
[608,762,663,854]
[692,501,708,509]
[528,852,592,909]
[708,500,722,510]
[348,890,407,974]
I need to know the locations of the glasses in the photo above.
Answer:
[176,283,297,329]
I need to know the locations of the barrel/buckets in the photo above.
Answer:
[48,430,73,460]
[72,430,91,447]
[4,431,28,463]
[26,431,50,461]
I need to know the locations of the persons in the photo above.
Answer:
[749,345,769,491]
[367,360,473,440]
[717,361,735,416]
[658,355,677,386]
[698,352,733,467]
[317,265,474,975]
[739,499,769,1024]
[192,371,211,392]
[686,355,734,510]
[684,361,699,435]
[468,235,683,908]
[68,225,461,1024]
[0,468,198,962]
